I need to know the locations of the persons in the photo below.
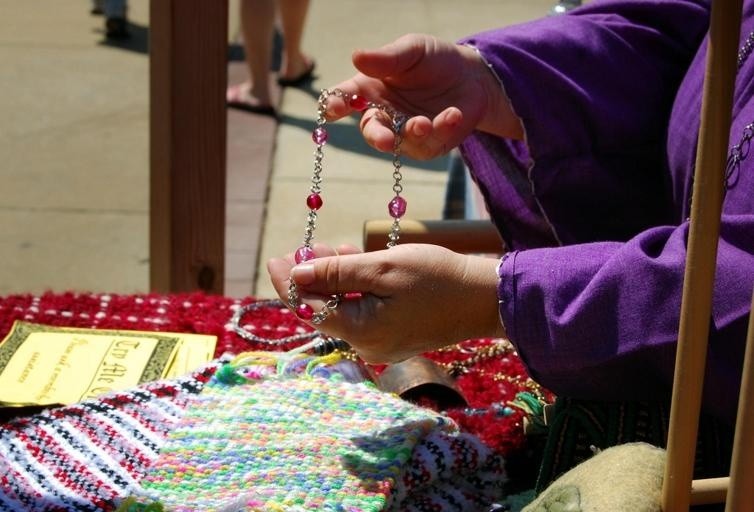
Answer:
[225,1,316,114]
[89,0,132,46]
[282,0,752,512]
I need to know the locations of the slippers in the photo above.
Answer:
[279,58,314,86]
[226,84,275,114]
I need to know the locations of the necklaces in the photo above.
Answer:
[687,34,751,223]
[287,83,408,324]
[225,299,552,422]
